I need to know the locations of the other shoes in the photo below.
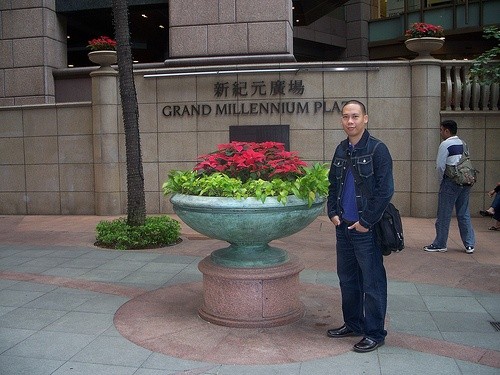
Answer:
[488,225,500,230]
[480,210,495,218]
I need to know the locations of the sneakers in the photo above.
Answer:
[466,246,474,254]
[423,244,448,252]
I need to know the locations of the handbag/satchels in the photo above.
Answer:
[373,202,405,256]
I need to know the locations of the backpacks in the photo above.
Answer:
[444,143,480,186]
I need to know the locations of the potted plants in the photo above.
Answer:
[403,21,446,59]
[160,141,328,270]
[86,36,117,71]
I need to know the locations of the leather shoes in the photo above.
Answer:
[352,336,384,351]
[327,323,363,337]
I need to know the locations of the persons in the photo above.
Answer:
[423,120,476,253]
[479,182,500,231]
[327,100,394,353]
